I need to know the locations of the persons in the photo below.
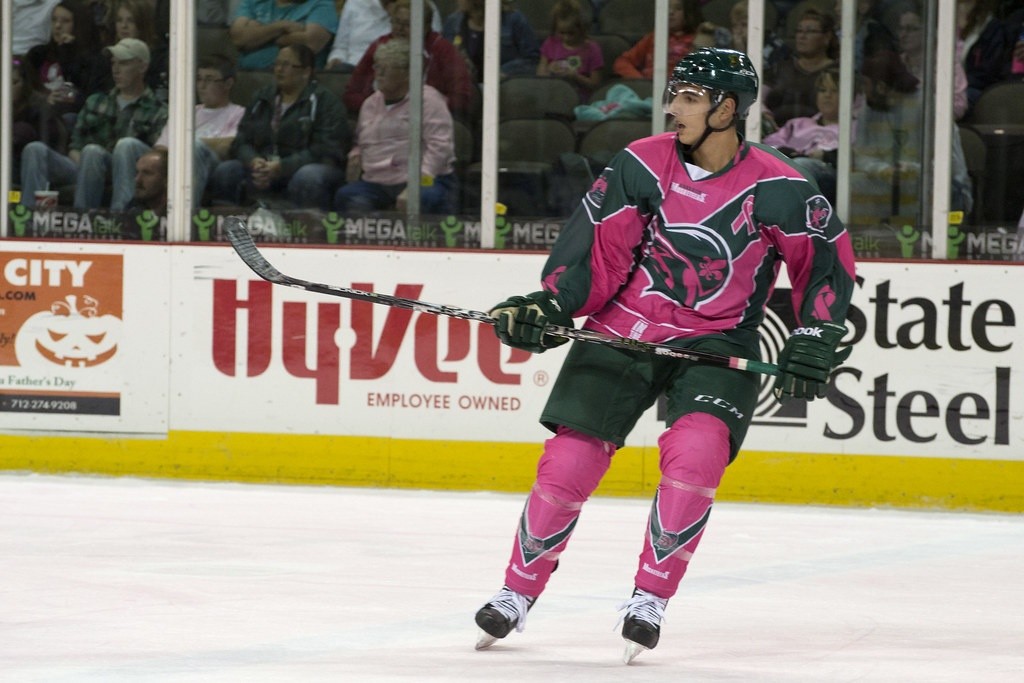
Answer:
[13,1,59,61]
[343,0,466,116]
[112,57,243,207]
[611,0,696,76]
[760,7,839,123]
[210,40,344,209]
[20,37,167,212]
[729,1,788,78]
[957,2,1011,99]
[825,0,888,56]
[124,149,166,211]
[324,0,443,75]
[686,20,737,49]
[24,2,96,128]
[472,50,855,659]
[851,53,973,225]
[336,43,459,215]
[536,1,603,87]
[10,60,60,190]
[892,19,968,118]
[443,0,534,86]
[87,1,164,89]
[219,1,338,71]
[765,64,837,193]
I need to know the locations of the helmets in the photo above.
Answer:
[667,46,760,122]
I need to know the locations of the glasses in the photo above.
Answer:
[272,58,306,70]
[373,62,408,73]
[196,76,233,85]
[895,24,922,34]
[389,18,410,29]
[795,27,824,35]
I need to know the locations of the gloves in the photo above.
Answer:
[769,318,848,404]
[487,288,575,354]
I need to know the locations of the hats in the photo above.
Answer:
[102,37,151,62]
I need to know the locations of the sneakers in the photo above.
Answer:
[618,585,672,664]
[469,585,537,650]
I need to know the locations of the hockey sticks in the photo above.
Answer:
[217,213,785,380]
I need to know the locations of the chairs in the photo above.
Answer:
[497,75,584,134]
[561,118,654,216]
[468,119,578,213]
